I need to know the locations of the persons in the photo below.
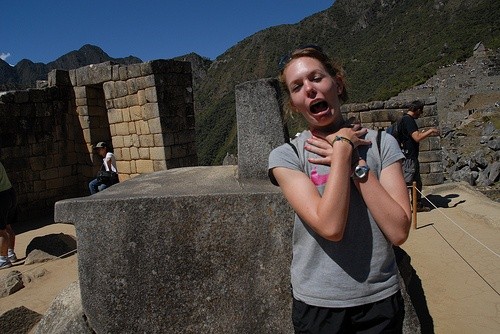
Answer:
[392,101,440,212]
[265,44,412,334]
[0,163,18,269]
[88,142,119,195]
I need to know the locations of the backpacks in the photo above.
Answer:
[386,115,412,139]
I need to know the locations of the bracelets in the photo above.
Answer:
[331,136,355,150]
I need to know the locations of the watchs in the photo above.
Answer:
[350,164,370,181]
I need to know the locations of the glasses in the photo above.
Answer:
[279,44,324,76]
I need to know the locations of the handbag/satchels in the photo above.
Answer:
[97,170,117,186]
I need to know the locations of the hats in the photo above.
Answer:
[93,142,106,150]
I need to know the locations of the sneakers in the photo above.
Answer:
[7,253,17,263]
[0,256,12,268]
